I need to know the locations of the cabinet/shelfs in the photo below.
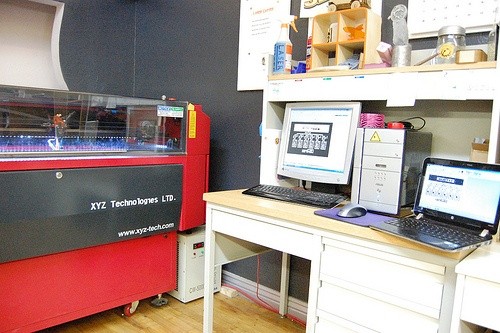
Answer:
[310,8,381,71]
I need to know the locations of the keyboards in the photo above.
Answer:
[242,184,349,210]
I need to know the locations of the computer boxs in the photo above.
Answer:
[351,129,433,215]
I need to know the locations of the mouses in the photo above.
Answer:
[336,203,367,218]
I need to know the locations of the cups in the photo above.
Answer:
[471,143,489,163]
[392,44,412,67]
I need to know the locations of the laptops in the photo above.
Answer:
[369,156,500,251]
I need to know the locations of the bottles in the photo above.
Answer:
[273,23,292,75]
[436,25,466,64]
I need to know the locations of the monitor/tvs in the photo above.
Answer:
[278,101,362,194]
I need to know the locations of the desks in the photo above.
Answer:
[202,181,500,333]
